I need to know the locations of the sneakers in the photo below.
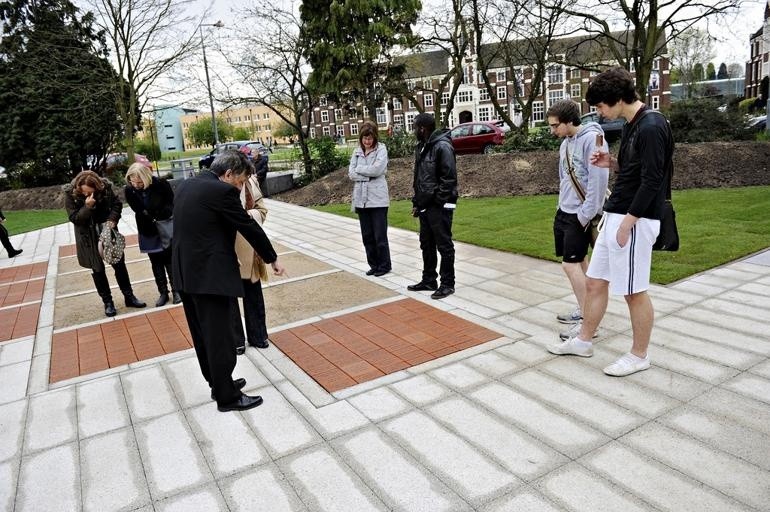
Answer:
[550,339,596,359]
[559,310,587,322]
[604,354,653,376]
[560,329,585,339]
[410,279,435,292]
[213,378,246,398]
[433,284,455,299]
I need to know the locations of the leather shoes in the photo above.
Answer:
[250,342,270,348]
[173,288,180,305]
[367,270,378,276]
[219,396,262,412]
[376,270,390,277]
[237,345,245,356]
[153,285,168,307]
[5,250,24,257]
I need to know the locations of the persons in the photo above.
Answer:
[124,163,182,307]
[546,102,612,340]
[172,150,234,364]
[407,113,457,299]
[236,175,269,355]
[168,152,289,412]
[0,209,23,260]
[249,149,270,189]
[346,121,392,276]
[546,66,673,375]
[65,172,146,316]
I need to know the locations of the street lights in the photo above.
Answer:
[198,21,224,148]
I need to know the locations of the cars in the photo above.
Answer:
[580,111,626,143]
[451,122,505,154]
[67,153,153,174]
[198,141,269,169]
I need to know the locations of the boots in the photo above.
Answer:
[113,272,148,307]
[91,268,117,315]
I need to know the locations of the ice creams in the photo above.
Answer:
[596,134,603,152]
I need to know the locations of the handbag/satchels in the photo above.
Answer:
[99,217,126,266]
[564,139,611,247]
[151,213,172,252]
[620,110,679,251]
